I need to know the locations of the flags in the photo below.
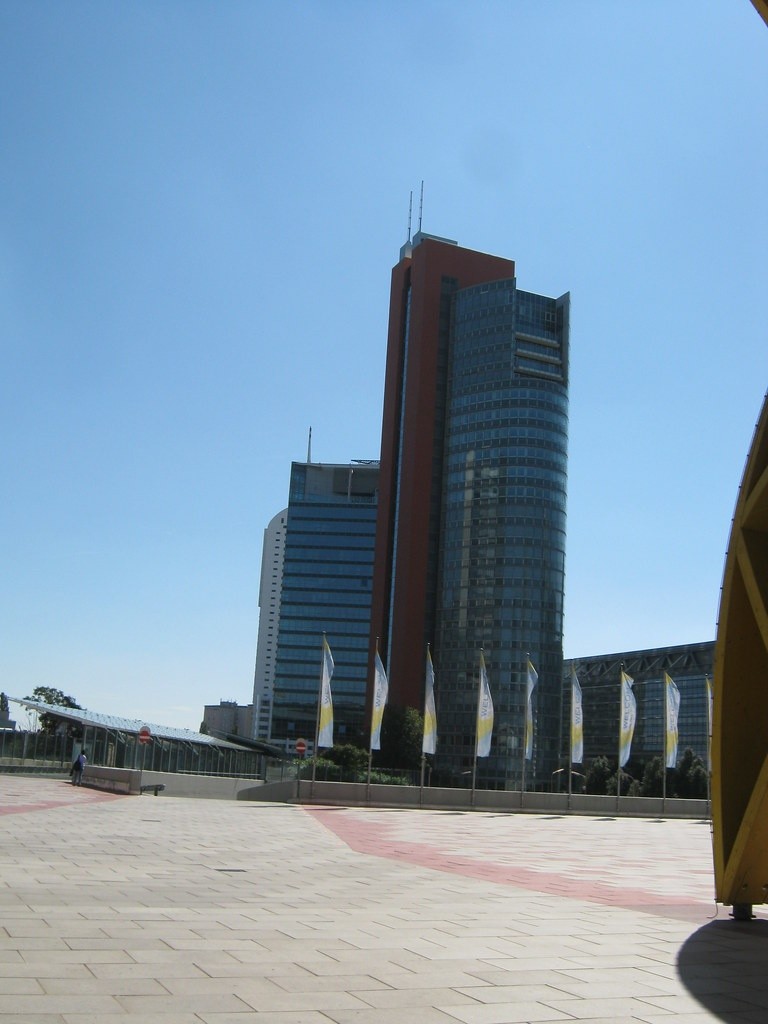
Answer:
[664,672,680,767]
[704,677,712,776]
[422,651,438,754]
[619,671,636,767]
[369,649,388,750]
[477,655,494,757]
[318,639,334,747]
[525,661,538,760]
[570,664,583,763]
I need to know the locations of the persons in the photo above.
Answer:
[72,749,86,786]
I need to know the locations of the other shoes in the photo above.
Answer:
[71,782,75,786]
[77,783,81,787]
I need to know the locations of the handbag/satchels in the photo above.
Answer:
[73,755,81,771]
